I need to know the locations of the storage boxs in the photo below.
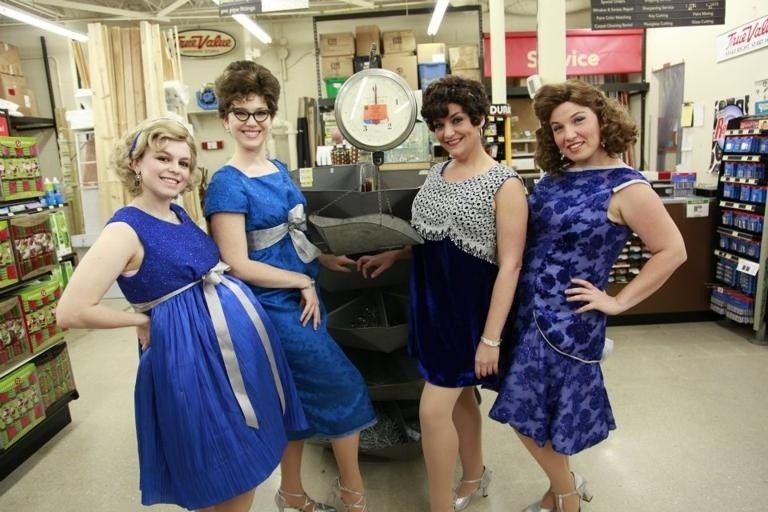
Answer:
[319,25,485,100]
[1,39,40,117]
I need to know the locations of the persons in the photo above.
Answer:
[356,76,531,512]
[481,81,688,512]
[202,59,380,510]
[56,118,310,511]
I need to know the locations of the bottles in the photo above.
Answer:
[43,177,57,206]
[51,177,64,206]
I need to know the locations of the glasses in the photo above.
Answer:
[228,107,272,122]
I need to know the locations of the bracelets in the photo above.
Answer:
[295,273,317,290]
[479,335,503,349]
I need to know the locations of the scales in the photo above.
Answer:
[308,43,426,257]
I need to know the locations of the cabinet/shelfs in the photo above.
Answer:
[0,107,79,480]
